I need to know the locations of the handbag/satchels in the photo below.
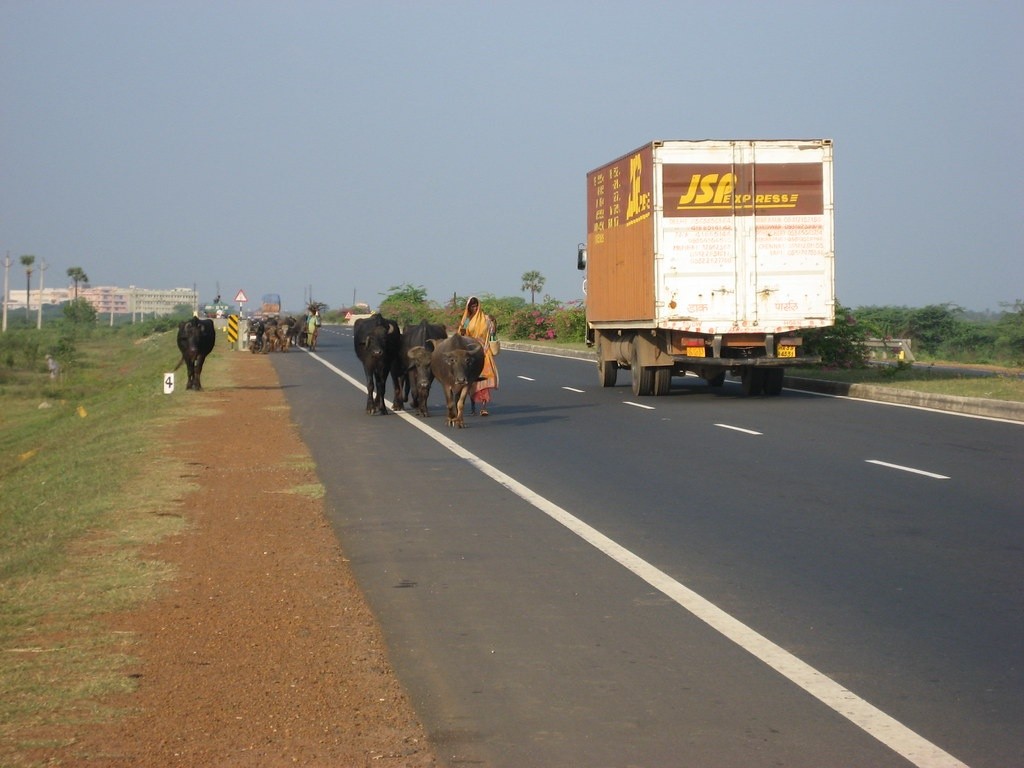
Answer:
[488,332,500,356]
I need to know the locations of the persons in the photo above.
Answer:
[458,297,496,415]
[307,308,318,351]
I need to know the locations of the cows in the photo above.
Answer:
[354,312,485,428]
[172,316,216,391]
[246,314,309,354]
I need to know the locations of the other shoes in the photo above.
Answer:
[480,409,488,416]
[471,409,477,417]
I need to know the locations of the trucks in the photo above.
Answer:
[576,138,834,398]
